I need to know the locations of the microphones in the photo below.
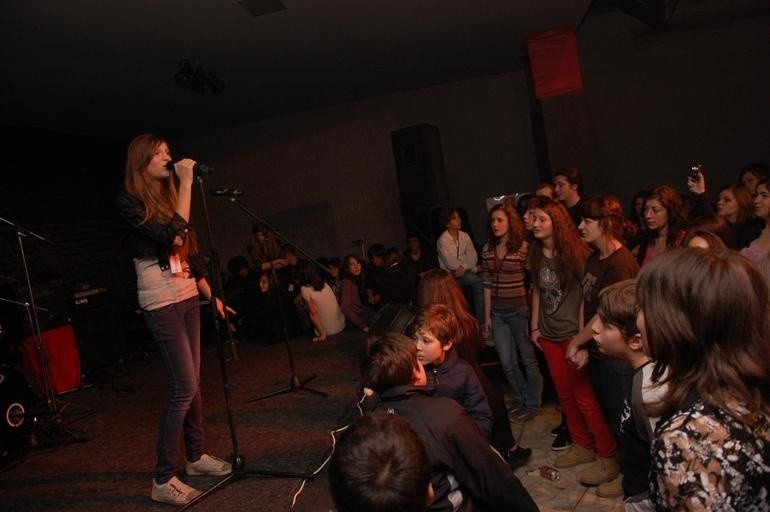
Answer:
[166,161,212,175]
[209,188,242,196]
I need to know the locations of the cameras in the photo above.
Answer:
[690,162,703,183]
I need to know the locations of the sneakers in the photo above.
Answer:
[151,476,202,506]
[183,454,232,476]
[507,405,536,425]
[551,432,573,451]
[550,424,568,437]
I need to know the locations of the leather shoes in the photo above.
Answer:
[579,456,619,485]
[554,443,597,468]
[594,473,625,499]
[502,445,532,463]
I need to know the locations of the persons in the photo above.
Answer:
[554,165,588,225]
[639,183,693,270]
[435,205,497,351]
[681,211,738,244]
[418,265,534,470]
[739,177,770,278]
[564,192,651,502]
[325,259,344,304]
[686,158,770,247]
[406,231,436,295]
[405,301,494,445]
[590,276,673,510]
[291,257,346,343]
[527,193,621,486]
[233,273,284,342]
[680,228,731,252]
[365,243,402,283]
[532,181,554,199]
[248,222,315,283]
[387,245,402,275]
[364,282,413,311]
[480,200,543,421]
[117,130,238,508]
[360,330,544,512]
[223,256,254,345]
[340,253,419,336]
[523,197,581,452]
[715,183,766,249]
[327,408,438,512]
[634,245,770,512]
[515,193,530,220]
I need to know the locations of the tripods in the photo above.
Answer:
[2,220,94,443]
[172,180,314,512]
[234,200,330,408]
